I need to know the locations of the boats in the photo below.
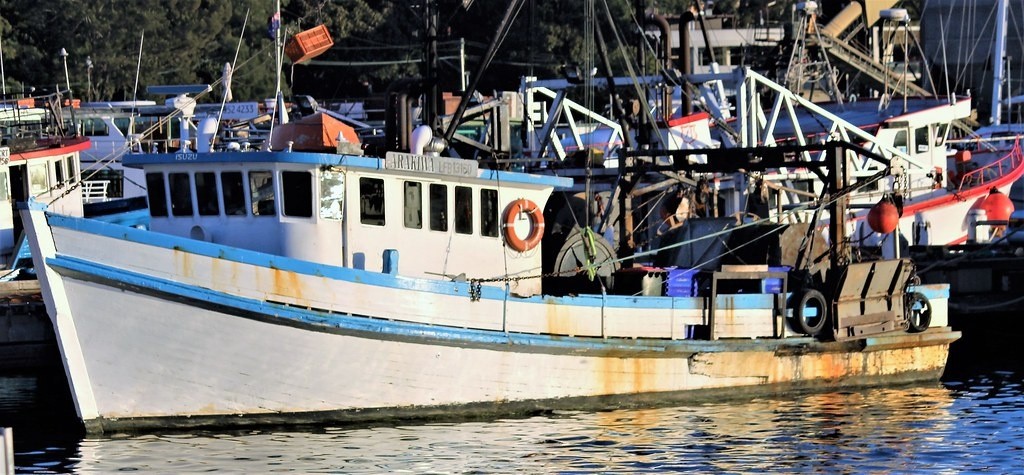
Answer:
[0,0,1024,439]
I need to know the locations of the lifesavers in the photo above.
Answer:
[903,292,932,332]
[790,289,828,333]
[502,199,546,252]
